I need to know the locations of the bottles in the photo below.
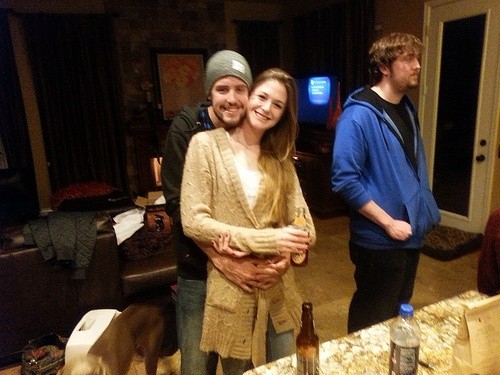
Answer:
[388,303,420,375]
[295,302,319,375]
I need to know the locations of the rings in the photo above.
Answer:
[232,251,235,256]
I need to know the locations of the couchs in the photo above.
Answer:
[0,212,125,368]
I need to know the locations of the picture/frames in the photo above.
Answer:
[150,47,209,123]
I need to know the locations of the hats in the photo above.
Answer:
[204,50,253,96]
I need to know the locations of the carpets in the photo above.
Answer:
[421,224,483,262]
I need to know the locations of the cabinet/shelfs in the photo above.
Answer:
[292,151,341,220]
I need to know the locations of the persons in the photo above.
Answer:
[181,70,316,375]
[160,50,290,375]
[332,30,442,333]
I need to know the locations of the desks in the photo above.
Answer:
[237,289,490,375]
[120,242,178,356]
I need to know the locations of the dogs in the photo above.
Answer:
[56,282,179,375]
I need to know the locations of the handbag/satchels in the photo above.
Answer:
[21,345,64,375]
